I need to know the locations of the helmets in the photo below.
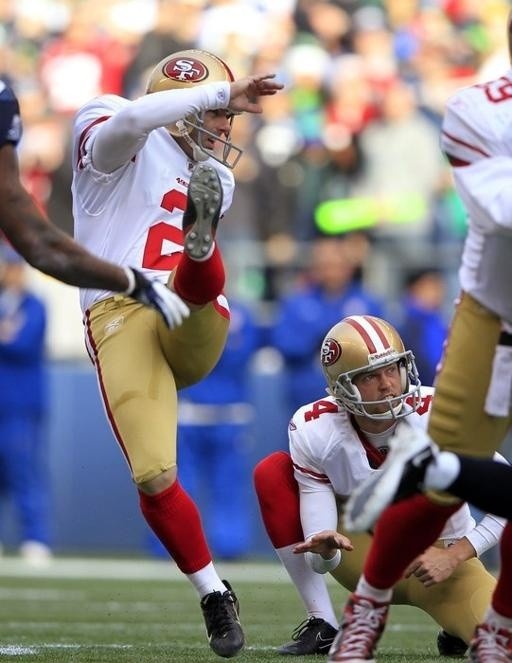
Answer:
[319,314,422,422]
[144,49,244,169]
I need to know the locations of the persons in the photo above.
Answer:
[0,0,511,564]
[327,9,512,663]
[253,316,512,657]
[72,50,284,658]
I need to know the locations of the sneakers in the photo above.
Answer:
[436,629,469,659]
[200,579,246,659]
[469,623,512,663]
[343,424,441,532]
[181,161,223,260]
[275,615,338,656]
[325,592,391,663]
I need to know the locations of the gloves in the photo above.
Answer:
[117,265,191,333]
